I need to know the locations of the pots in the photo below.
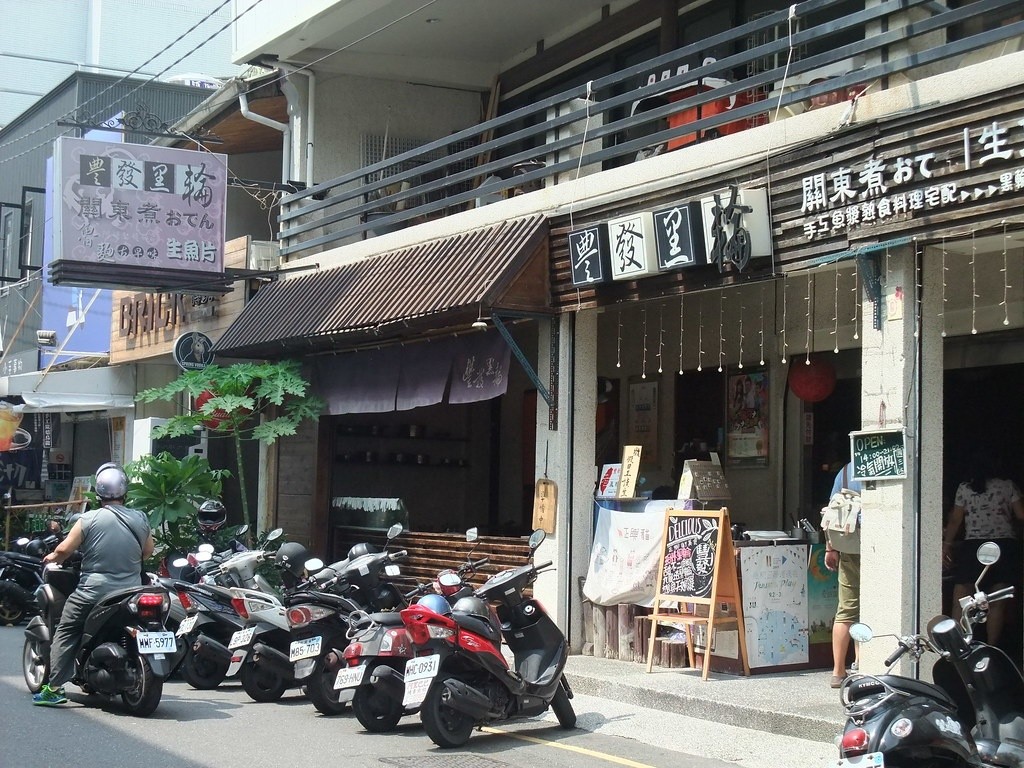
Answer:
[335,449,378,466]
[336,423,427,439]
[386,451,430,466]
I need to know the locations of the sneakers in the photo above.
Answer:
[32,683,67,705]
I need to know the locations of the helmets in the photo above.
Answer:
[95,462,128,499]
[417,594,453,616]
[348,542,379,562]
[25,539,50,558]
[455,595,490,622]
[198,499,227,533]
[274,542,309,578]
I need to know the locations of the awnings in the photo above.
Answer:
[206,214,554,359]
[0,363,137,413]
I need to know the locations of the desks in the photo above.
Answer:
[698,540,856,675]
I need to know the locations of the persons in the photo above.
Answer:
[824,462,860,689]
[33,462,155,705]
[943,445,1024,646]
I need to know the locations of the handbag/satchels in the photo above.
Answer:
[140,569,151,585]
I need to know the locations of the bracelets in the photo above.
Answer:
[825,549,836,552]
[944,541,953,545]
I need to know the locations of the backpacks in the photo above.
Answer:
[820,464,861,554]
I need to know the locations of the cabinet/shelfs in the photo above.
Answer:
[334,423,470,466]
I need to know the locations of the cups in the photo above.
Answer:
[790,527,808,541]
[807,529,824,545]
[700,442,708,452]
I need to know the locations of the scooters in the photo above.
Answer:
[832,542,1024,768]
[401,529,577,749]
[332,527,492,731]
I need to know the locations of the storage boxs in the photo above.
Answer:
[666,85,748,152]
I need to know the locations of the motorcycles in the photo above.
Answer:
[0,515,350,701]
[23,551,173,718]
[284,522,410,717]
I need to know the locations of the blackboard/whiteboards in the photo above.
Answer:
[850,427,908,482]
[656,508,740,605]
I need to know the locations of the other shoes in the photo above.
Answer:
[830,673,852,687]
[850,662,859,673]
